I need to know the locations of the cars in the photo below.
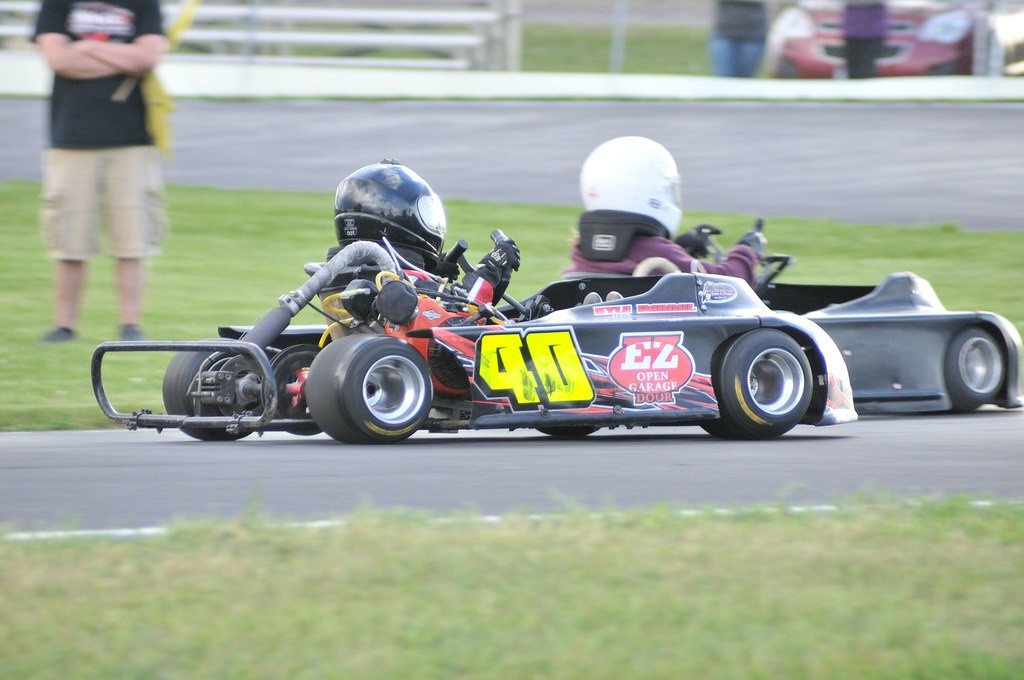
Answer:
[775,0,1024,79]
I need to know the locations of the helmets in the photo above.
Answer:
[580,136,682,241]
[334,162,446,260]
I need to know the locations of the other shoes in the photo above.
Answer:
[121,327,144,341]
[47,325,71,342]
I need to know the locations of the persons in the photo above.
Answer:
[843,0,888,78]
[31,0,169,342]
[565,136,771,291]
[707,0,768,77]
[326,163,521,324]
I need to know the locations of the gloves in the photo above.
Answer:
[482,238,520,269]
[432,253,460,285]
[675,224,721,259]
[739,230,765,254]
